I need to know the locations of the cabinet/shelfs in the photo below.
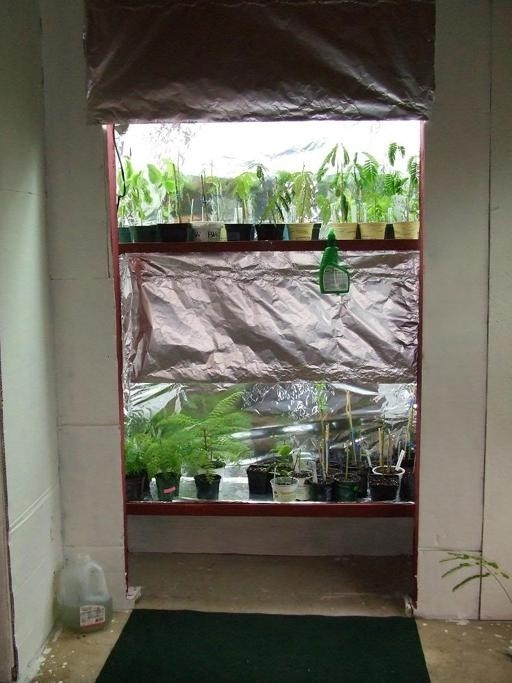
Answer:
[99,113,424,557]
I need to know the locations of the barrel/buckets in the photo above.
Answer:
[55,555,112,634]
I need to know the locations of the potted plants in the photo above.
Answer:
[124,384,418,502]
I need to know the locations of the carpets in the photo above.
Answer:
[94,607,432,683]
